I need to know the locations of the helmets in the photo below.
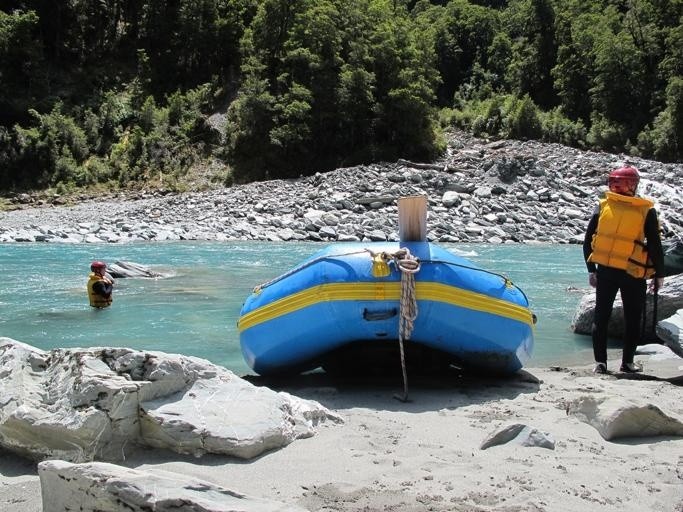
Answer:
[91,262,107,272]
[609,167,640,193]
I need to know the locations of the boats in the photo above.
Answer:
[230,236,538,390]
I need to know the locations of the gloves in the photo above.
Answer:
[589,272,597,288]
[649,278,663,292]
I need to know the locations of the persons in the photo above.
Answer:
[582,167,666,374]
[85,261,114,309]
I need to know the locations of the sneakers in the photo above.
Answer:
[592,362,606,373]
[620,363,640,372]
